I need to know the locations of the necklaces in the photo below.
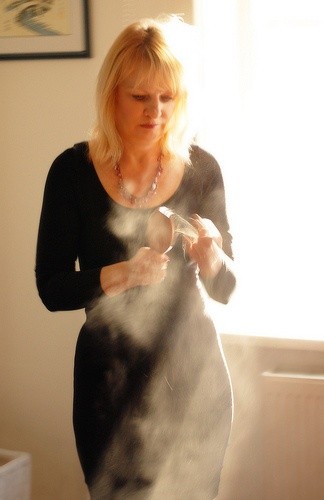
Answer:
[110,150,166,207]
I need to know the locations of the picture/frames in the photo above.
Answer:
[0,0,91,60]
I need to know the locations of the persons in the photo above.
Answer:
[36,22,236,500]
